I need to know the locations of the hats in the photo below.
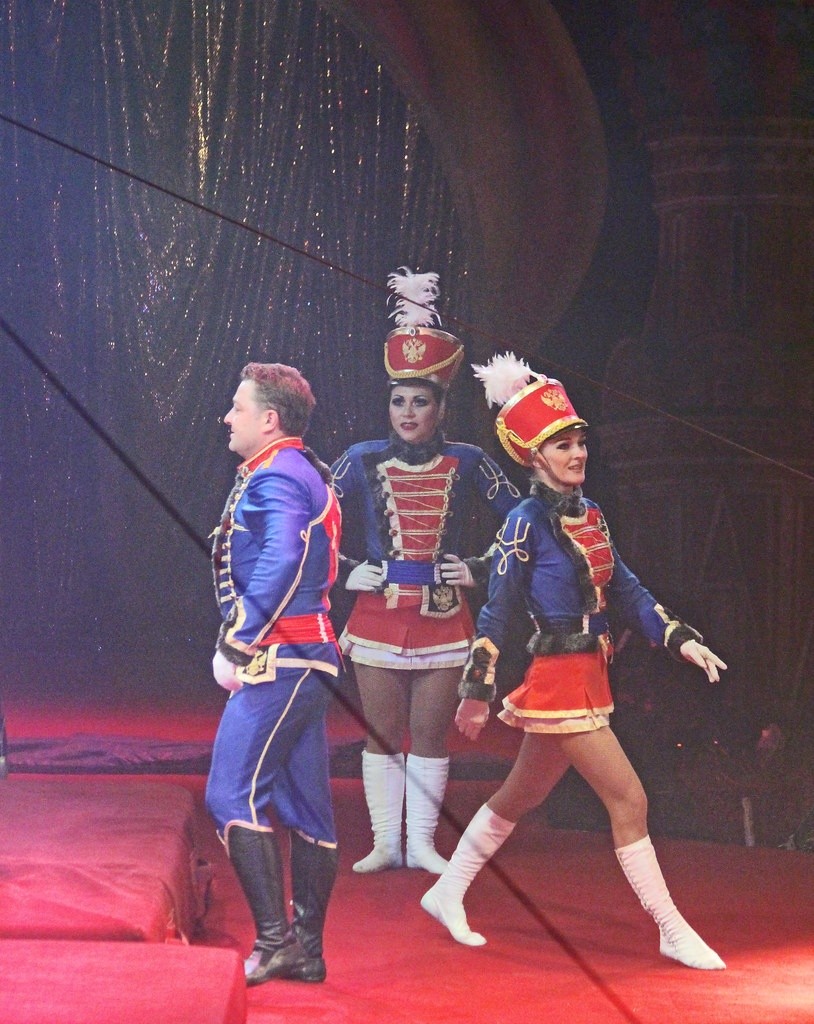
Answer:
[384,265,465,393]
[471,350,588,468]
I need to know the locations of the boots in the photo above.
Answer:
[420,803,516,946]
[278,827,339,983]
[406,754,449,874]
[614,835,726,970]
[215,818,301,984]
[352,750,404,874]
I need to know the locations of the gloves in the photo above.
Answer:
[345,559,384,591]
[455,697,489,743]
[679,639,728,682]
[440,554,477,588]
[212,650,244,691]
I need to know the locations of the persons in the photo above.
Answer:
[207,364,343,986]
[330,328,526,874]
[420,379,729,971]
